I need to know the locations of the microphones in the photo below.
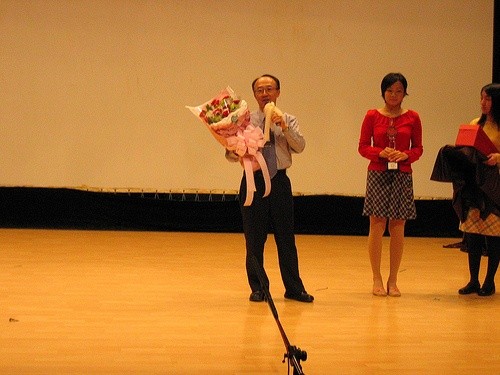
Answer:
[265,103,280,126]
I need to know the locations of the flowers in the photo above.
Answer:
[200,95,241,126]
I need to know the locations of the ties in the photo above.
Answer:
[261,127,277,180]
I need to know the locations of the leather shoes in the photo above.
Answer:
[249,291,264,301]
[285,290,314,302]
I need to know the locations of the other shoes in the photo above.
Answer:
[459,280,480,294]
[478,282,495,296]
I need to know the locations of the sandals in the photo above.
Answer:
[373,278,386,296]
[386,279,401,297]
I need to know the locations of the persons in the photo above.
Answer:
[459,84,500,296]
[226,74,314,302]
[358,72,423,297]
